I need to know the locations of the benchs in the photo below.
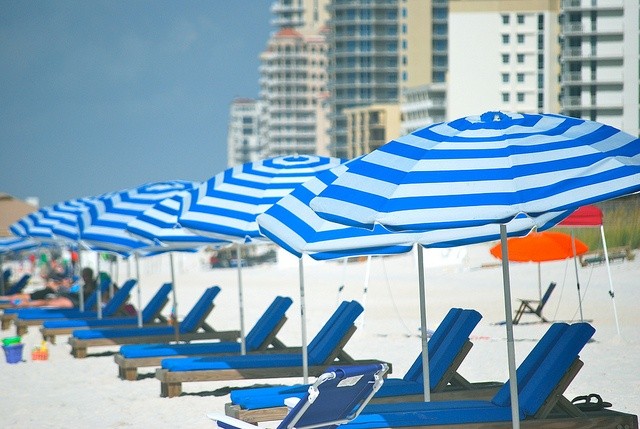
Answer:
[585,254,627,264]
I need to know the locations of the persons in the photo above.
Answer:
[0,259,67,302]
[17,251,25,268]
[28,251,37,274]
[39,247,48,268]
[9,267,97,310]
[50,249,61,260]
[69,249,80,267]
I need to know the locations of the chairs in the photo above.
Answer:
[515,280,556,324]
[1,272,113,330]
[212,309,506,425]
[16,278,137,334]
[40,282,173,348]
[114,296,294,386]
[152,301,392,398]
[216,364,387,429]
[332,323,638,428]
[67,286,228,358]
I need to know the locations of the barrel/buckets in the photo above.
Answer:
[1,344,23,363]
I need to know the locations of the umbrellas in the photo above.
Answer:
[308,110,640,429]
[80,183,232,343]
[9,190,91,318]
[176,151,349,386]
[70,188,125,326]
[0,233,58,296]
[490,231,588,309]
[125,185,246,356]
[256,153,578,404]
